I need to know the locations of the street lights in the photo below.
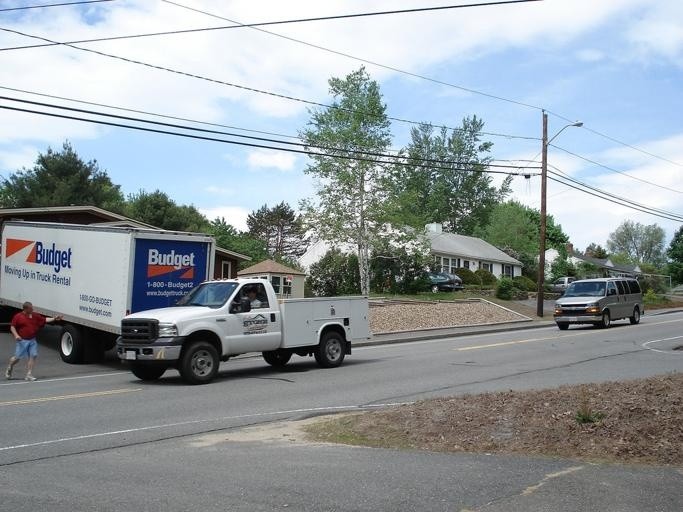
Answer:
[538,113,583,317]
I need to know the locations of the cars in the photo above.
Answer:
[397,271,465,294]
[542,276,576,295]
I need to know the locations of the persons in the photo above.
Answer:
[246,286,262,307]
[4,301,64,381]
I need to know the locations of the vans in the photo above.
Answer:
[553,277,644,329]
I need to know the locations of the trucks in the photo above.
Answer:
[0,218,216,364]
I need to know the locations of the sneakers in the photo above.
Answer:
[25,375,36,381]
[6,367,12,379]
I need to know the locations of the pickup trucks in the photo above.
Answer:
[116,277,372,383]
